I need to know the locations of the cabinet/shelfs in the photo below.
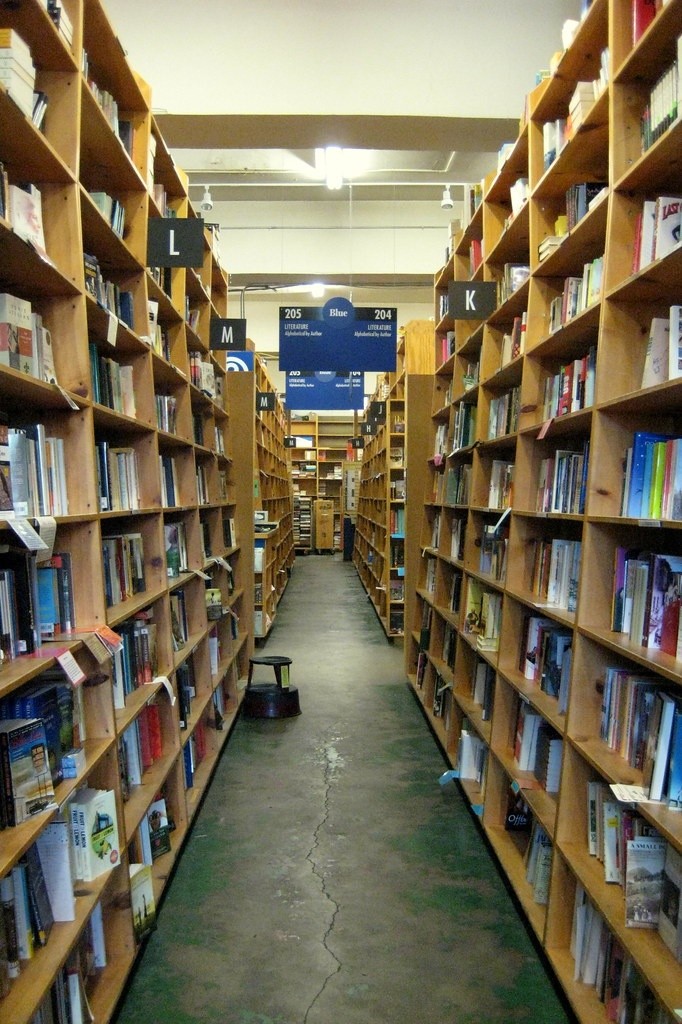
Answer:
[0,0,682,1024]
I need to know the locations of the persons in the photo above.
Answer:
[149,810,161,830]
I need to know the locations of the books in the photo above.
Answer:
[327,0,682,1023]
[0,0,325,1024]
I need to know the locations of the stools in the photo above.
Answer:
[242,655,302,719]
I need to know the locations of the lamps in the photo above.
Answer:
[440,186,454,208]
[200,184,214,211]
